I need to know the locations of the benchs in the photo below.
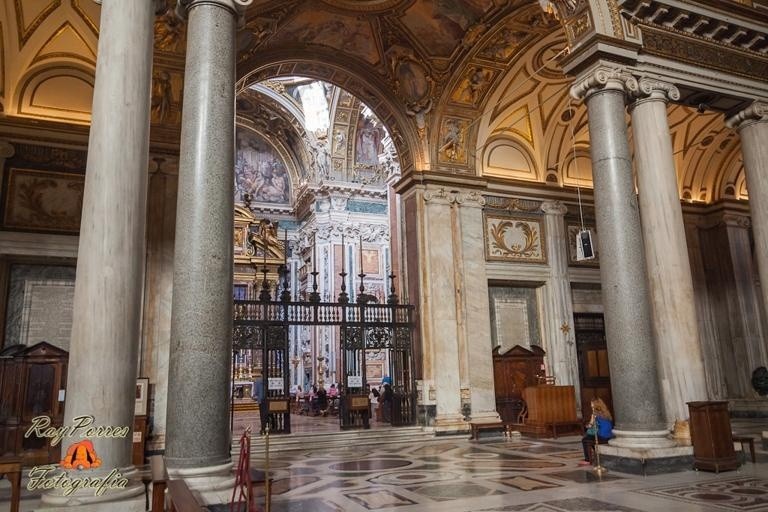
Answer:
[469,420,512,439]
[289,394,341,417]
[583,438,607,463]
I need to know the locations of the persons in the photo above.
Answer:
[382,384,392,423]
[369,388,379,422]
[235,154,288,203]
[257,371,266,434]
[296,384,337,417]
[578,398,612,466]
[158,72,175,126]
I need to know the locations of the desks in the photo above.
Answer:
[226,379,254,399]
[518,383,576,426]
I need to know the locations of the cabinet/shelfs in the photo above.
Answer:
[0,340,69,465]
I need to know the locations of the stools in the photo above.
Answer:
[732,437,755,463]
[0,456,22,512]
[232,468,273,512]
